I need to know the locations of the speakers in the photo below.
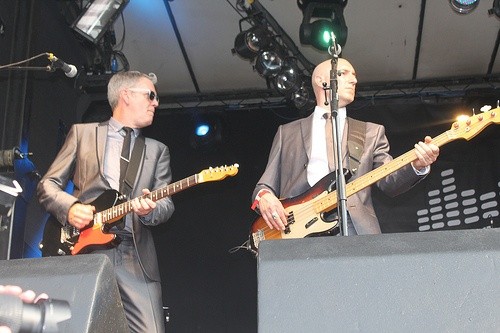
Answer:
[257,227,500,333]
[0,253,131,333]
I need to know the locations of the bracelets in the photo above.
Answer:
[253,190,271,215]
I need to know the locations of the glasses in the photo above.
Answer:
[128,88,160,105]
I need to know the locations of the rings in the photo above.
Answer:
[272,213,278,219]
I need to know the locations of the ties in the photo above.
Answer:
[113,127,133,230]
[325,112,338,172]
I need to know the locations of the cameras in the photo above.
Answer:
[0,294,72,333]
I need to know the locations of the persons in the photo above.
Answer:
[252,59,440,235]
[37,71,174,332]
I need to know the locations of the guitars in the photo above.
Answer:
[248,104,500,255]
[42,162,239,257]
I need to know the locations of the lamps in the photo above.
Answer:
[189,115,210,137]
[449,0,480,15]
[95,51,129,75]
[231,0,348,113]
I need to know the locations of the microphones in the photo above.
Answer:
[328,32,342,56]
[46,53,77,78]
